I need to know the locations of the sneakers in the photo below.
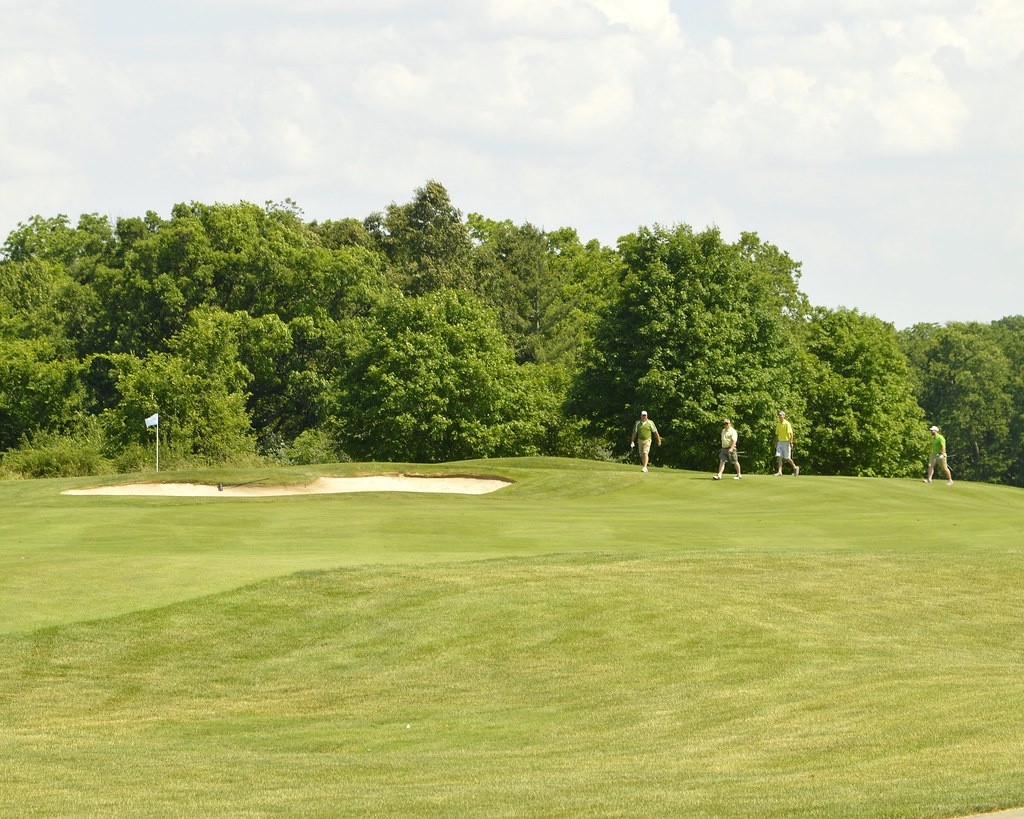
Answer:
[774,472,782,476]
[945,480,954,485]
[794,466,801,476]
[641,467,649,473]
[713,475,722,480]
[733,476,742,480]
[923,479,932,484]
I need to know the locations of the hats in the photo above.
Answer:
[723,420,730,425]
[929,426,939,432]
[642,410,648,416]
[778,410,785,416]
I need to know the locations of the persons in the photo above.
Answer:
[923,426,953,486]
[713,419,741,479]
[774,411,800,475]
[631,411,661,472]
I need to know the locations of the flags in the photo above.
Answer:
[145,413,158,427]
